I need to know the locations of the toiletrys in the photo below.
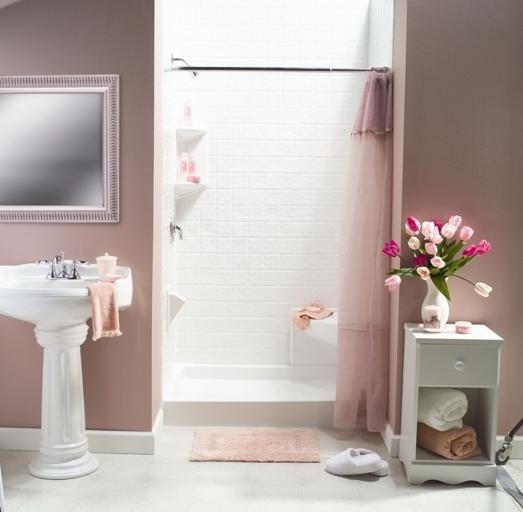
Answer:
[177,150,198,184]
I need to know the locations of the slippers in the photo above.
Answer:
[326,447,389,476]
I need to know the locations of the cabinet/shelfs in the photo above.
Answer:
[397,321,504,486]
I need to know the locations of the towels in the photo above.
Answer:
[416,424,481,460]
[291,299,335,331]
[85,277,123,343]
[417,388,469,433]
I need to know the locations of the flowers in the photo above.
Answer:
[382,214,493,299]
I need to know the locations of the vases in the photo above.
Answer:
[419,275,451,333]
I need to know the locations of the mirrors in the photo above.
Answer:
[0,73,122,225]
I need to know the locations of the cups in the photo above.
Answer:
[95,252,118,281]
[454,319,471,335]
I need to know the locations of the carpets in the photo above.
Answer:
[187,428,321,464]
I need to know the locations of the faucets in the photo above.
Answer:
[52,249,68,265]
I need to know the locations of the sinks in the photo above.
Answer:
[0,261,132,323]
